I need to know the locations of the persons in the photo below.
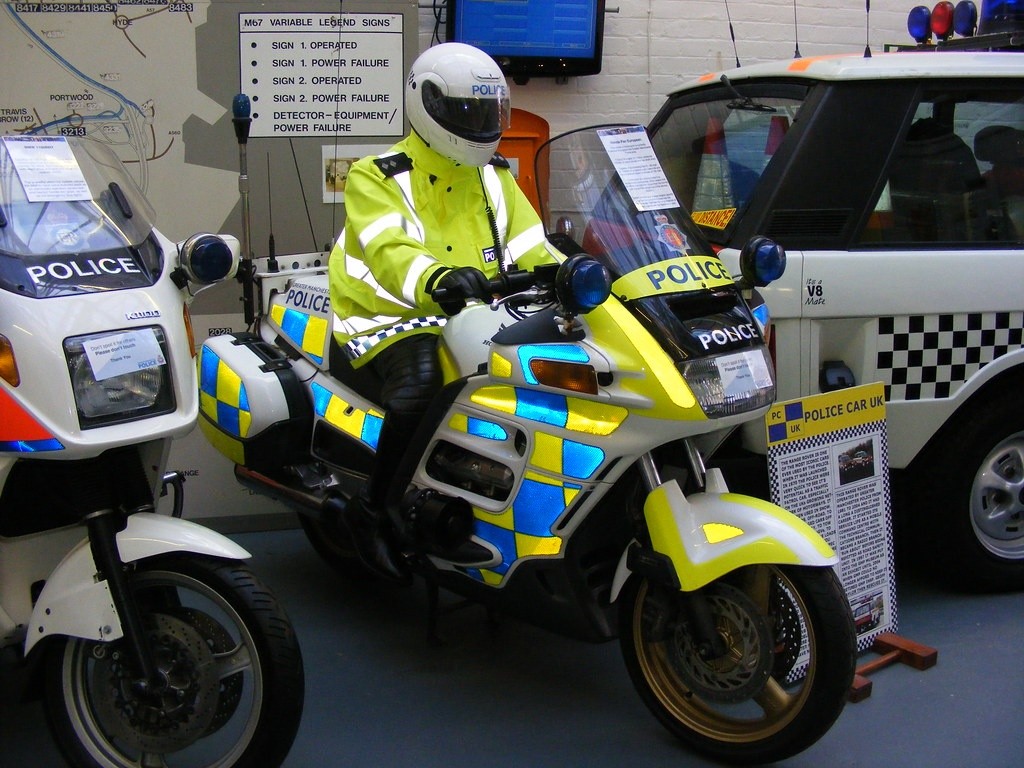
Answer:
[333,43,575,588]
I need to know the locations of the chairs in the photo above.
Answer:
[885,117,997,242]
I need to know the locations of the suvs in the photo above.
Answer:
[580,0,1024,596]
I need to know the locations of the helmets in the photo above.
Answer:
[404,41,510,168]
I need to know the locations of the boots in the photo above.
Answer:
[367,409,419,551]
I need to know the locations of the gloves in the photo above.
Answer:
[437,267,496,316]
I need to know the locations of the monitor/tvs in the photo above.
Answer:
[445,0,606,85]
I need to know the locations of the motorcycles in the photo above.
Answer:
[196,95,858,768]
[0,136,304,768]
